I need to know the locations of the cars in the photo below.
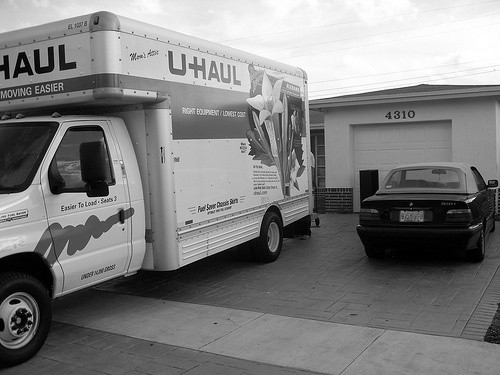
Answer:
[353,161,499,262]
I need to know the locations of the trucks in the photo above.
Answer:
[0,12,316,371]
